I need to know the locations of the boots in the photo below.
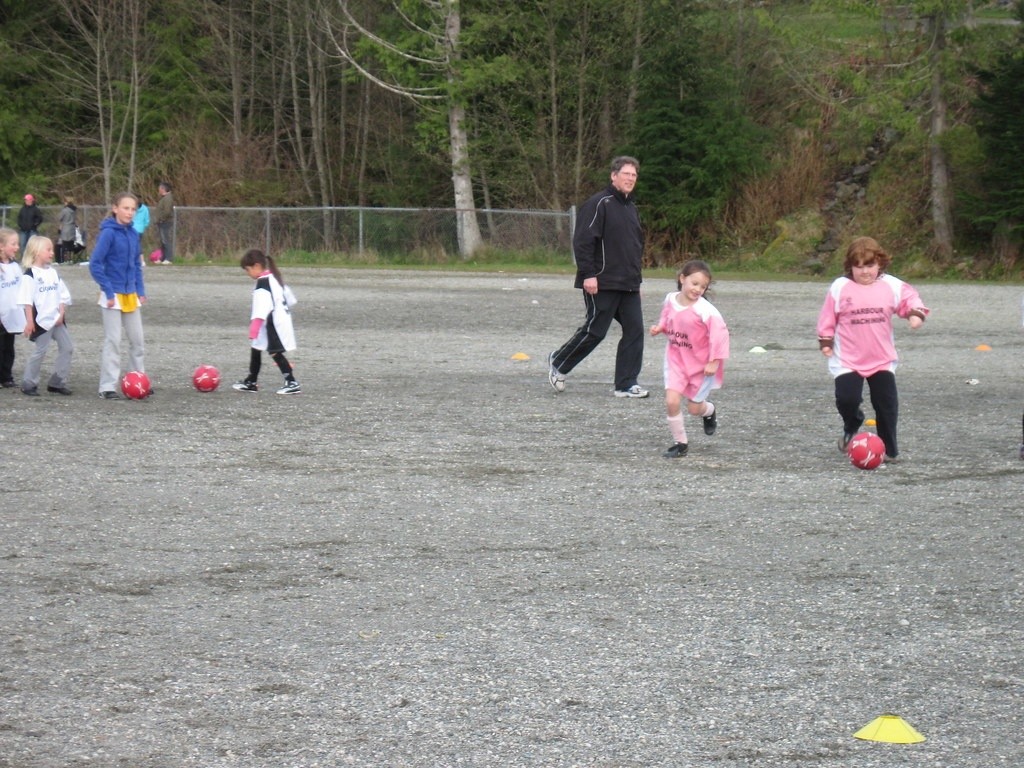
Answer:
[60,251,74,264]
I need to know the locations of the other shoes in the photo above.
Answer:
[838,431,858,451]
[884,455,900,462]
[3,382,20,388]
[21,388,38,396]
[47,385,72,395]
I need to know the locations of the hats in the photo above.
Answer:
[24,195,34,200]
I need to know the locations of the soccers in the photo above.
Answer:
[848,432,886,469]
[121,370,151,398]
[192,364,220,392]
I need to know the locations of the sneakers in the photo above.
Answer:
[161,259,173,264]
[148,389,155,396]
[276,380,302,393]
[704,401,717,435]
[664,441,689,456]
[99,391,119,399]
[548,351,566,392]
[232,380,259,392]
[614,384,649,397]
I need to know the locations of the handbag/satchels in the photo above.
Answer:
[283,285,297,308]
[151,248,163,260]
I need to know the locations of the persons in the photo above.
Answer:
[16,235,75,395]
[649,260,729,460]
[0,228,25,389]
[233,250,303,395]
[131,195,150,268]
[152,183,174,264]
[549,156,649,398]
[88,192,150,401]
[816,237,931,462]
[16,194,44,257]
[57,197,85,264]
